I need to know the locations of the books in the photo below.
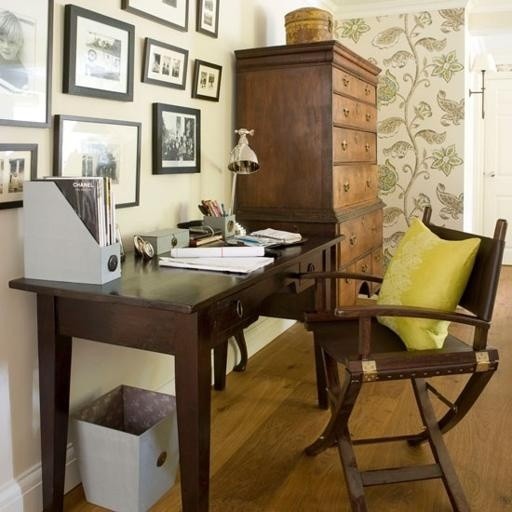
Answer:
[159,227,303,273]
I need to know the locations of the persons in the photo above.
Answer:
[90,38,113,49]
[162,131,194,160]
[80,151,116,182]
[0,10,28,89]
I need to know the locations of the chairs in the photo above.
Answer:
[298,207,507,512]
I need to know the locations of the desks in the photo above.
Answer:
[7,235,347,512]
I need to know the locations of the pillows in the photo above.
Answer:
[374,217,482,350]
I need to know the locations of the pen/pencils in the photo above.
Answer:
[238,238,259,243]
[198,200,229,218]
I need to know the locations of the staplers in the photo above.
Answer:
[177,220,205,234]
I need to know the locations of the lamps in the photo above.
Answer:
[229,128,261,214]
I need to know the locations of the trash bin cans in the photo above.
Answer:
[73,384,179,512]
